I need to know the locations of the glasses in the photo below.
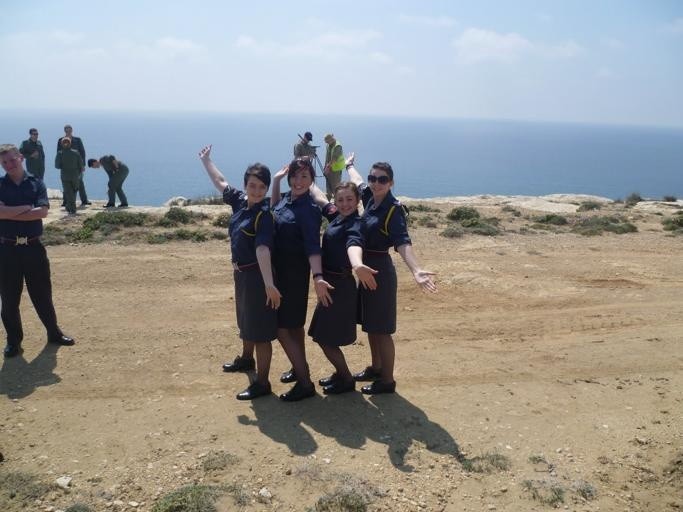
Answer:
[367,174,391,185]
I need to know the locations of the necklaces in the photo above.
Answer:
[371,202,379,210]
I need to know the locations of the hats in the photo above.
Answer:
[305,132,312,141]
[324,132,334,140]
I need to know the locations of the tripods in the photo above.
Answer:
[310,154,336,199]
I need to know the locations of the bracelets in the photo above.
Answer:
[312,272,323,280]
[344,163,353,171]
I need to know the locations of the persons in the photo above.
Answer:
[0,143,74,357]
[53,136,85,217]
[307,179,380,397]
[268,156,334,404]
[85,152,129,211]
[292,132,316,163]
[195,141,284,402]
[18,126,45,184]
[342,149,439,395]
[56,125,92,207]
[321,132,346,204]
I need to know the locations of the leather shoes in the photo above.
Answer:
[279,367,297,383]
[319,373,336,386]
[323,379,356,395]
[361,380,396,394]
[61,201,128,215]
[280,383,315,402]
[236,380,272,400]
[4,341,21,357]
[47,333,74,345]
[351,365,382,381]
[222,354,255,371]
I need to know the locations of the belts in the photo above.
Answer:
[0,236,43,246]
